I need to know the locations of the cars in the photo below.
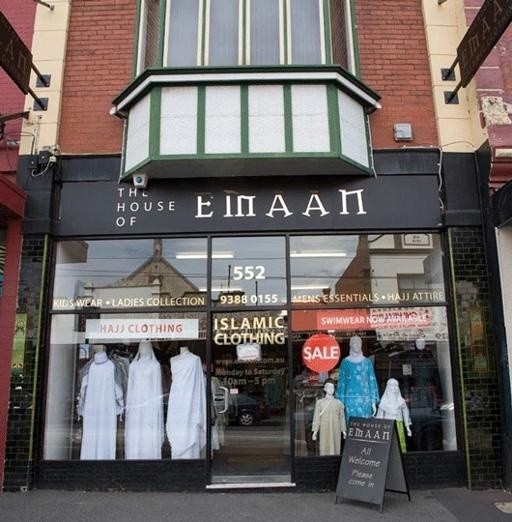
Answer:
[164,389,273,427]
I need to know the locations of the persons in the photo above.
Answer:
[334,336,381,418]
[165,346,208,460]
[375,378,413,454]
[312,378,347,457]
[123,341,165,461]
[76,351,124,461]
